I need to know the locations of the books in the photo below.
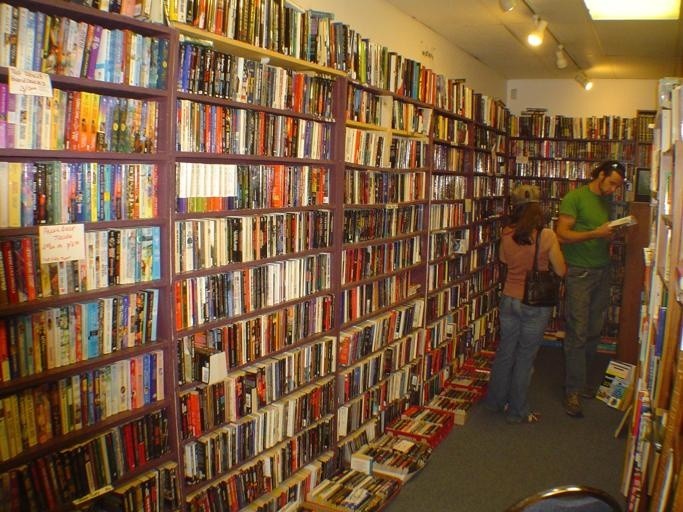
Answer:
[628,76,682,512]
[507,108,634,356]
[0,0,513,512]
[596,359,636,438]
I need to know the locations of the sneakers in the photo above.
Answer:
[564,385,596,415]
[502,403,542,423]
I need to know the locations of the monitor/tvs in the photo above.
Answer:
[635,168,651,202]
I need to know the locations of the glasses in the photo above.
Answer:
[602,163,625,171]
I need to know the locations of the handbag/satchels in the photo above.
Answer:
[522,271,559,307]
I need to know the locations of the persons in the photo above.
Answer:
[556,160,623,417]
[487,187,567,424]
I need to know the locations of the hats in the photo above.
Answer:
[511,184,541,205]
